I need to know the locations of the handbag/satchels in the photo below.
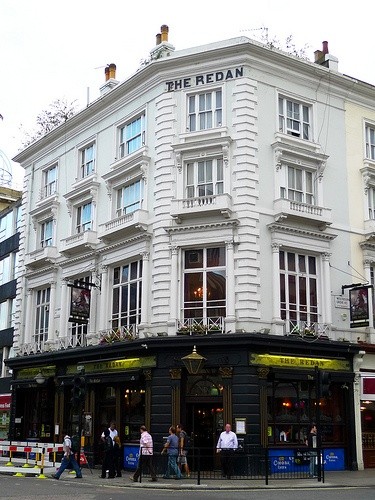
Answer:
[178,432,192,450]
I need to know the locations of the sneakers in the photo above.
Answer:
[75,476,82,478]
[51,474,59,480]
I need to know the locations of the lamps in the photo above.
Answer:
[33,370,49,384]
[181,344,208,375]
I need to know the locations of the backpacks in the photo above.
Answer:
[64,435,81,451]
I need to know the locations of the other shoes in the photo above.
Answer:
[148,478,156,482]
[99,474,122,479]
[186,475,190,477]
[129,476,138,482]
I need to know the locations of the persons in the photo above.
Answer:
[100,421,123,479]
[217,423,238,480]
[306,424,320,478]
[176,424,191,478]
[51,430,82,479]
[130,425,158,482]
[161,426,182,480]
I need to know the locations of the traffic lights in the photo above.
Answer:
[72,370,86,410]
